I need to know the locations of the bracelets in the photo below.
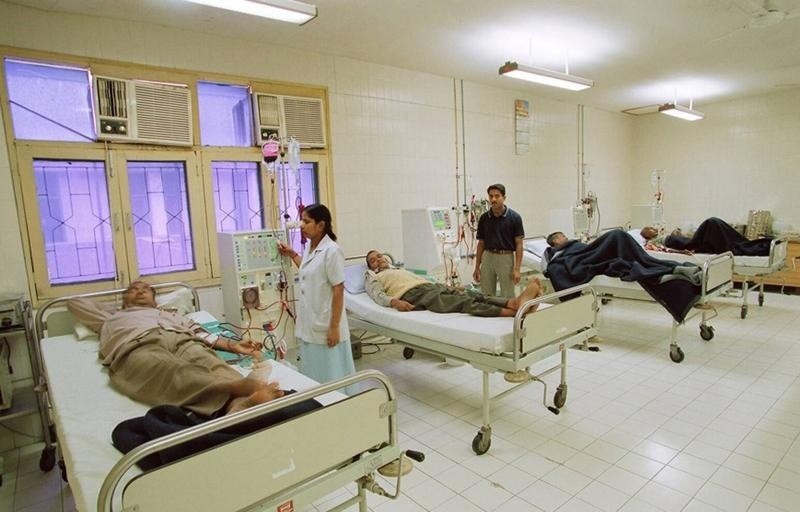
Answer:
[291,252,298,260]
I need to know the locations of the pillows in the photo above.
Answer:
[343,263,397,294]
[524,238,555,260]
[626,228,648,247]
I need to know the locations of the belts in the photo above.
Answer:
[485,247,513,254]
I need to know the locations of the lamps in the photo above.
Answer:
[197,0,319,26]
[497,38,594,95]
[659,90,706,123]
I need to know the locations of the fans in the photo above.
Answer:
[714,1,800,46]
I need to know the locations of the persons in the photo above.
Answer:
[472,183,524,300]
[362,250,543,318]
[640,217,770,256]
[544,227,686,276]
[275,203,358,395]
[66,281,284,422]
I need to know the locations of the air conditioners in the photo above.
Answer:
[250,90,328,149]
[93,74,194,147]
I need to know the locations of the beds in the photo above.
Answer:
[36,281,397,512]
[516,225,789,362]
[343,253,599,456]
